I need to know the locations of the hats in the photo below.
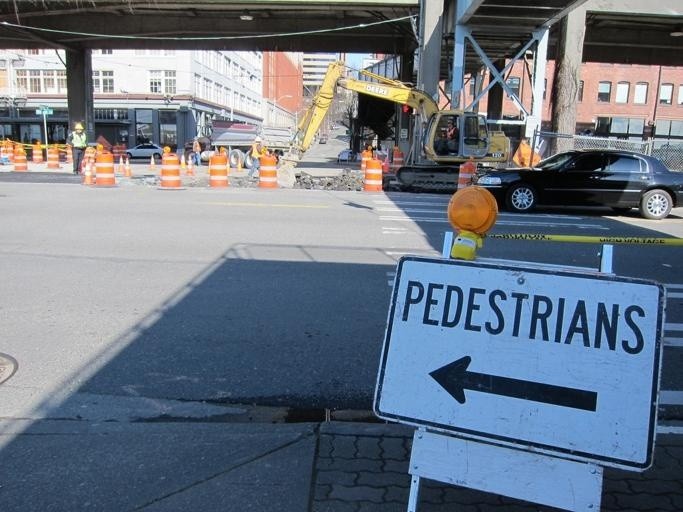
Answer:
[74,123,83,130]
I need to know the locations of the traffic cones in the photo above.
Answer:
[382,155,391,174]
[84,154,246,185]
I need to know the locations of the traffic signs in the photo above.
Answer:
[371,254,664,477]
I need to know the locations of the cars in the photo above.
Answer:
[467,145,682,220]
[126,142,164,159]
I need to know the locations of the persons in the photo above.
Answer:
[66,123,89,175]
[241,136,263,178]
[434,120,459,156]
[191,136,203,167]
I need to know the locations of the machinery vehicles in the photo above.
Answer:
[273,53,513,193]
